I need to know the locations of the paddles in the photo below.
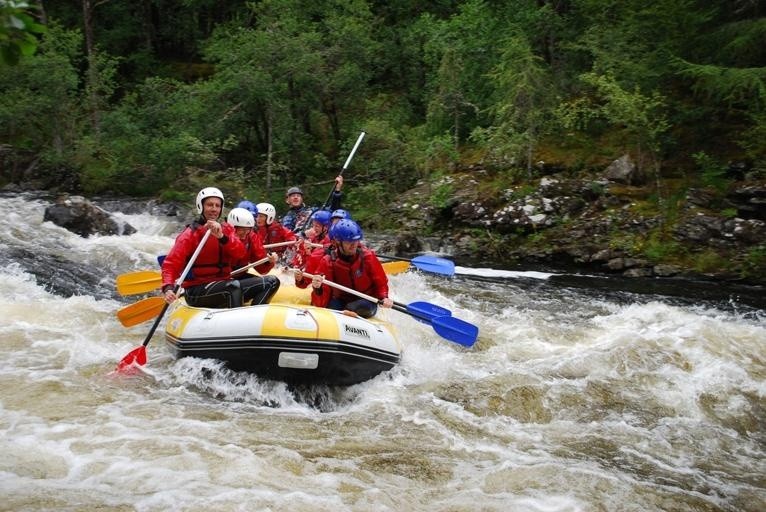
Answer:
[116,240,296,327]
[115,229,211,366]
[300,271,478,347]
[375,254,456,276]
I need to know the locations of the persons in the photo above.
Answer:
[161,175,395,319]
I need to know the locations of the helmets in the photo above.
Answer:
[227,200,276,228]
[311,209,363,241]
[196,187,225,216]
[286,187,304,197]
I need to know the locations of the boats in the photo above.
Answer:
[162,274,403,391]
